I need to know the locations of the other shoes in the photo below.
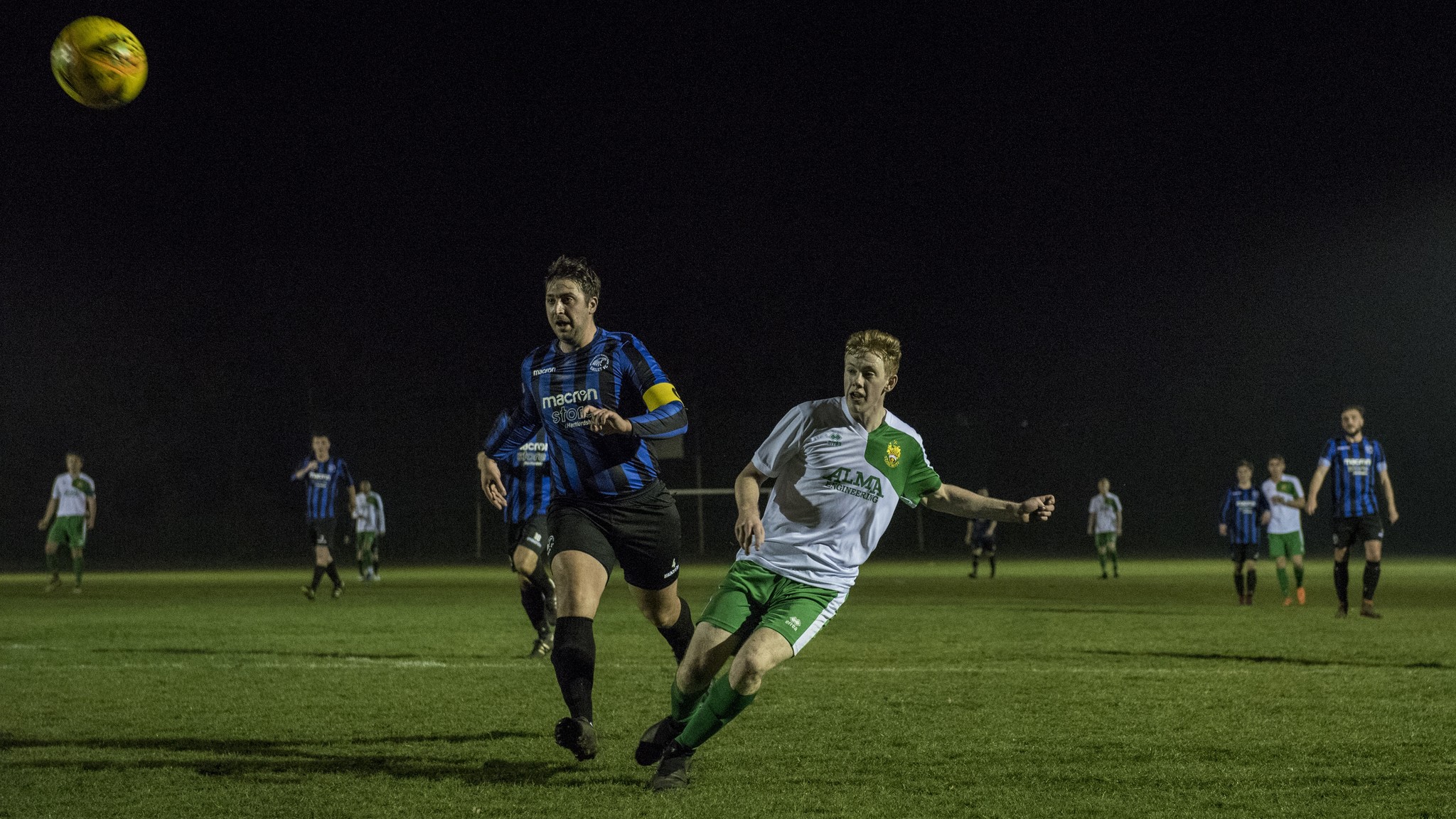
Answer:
[331,580,344,598]
[1334,603,1348,620]
[1245,594,1253,606]
[300,585,315,599]
[44,580,61,592]
[355,576,366,582]
[968,572,977,579]
[1098,573,1107,580]
[1114,572,1120,579]
[371,574,381,582]
[1360,601,1382,619]
[1238,596,1245,606]
[1296,586,1305,604]
[989,571,996,578]
[1283,597,1291,607]
[73,585,83,594]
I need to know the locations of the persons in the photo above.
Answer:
[291,428,358,603]
[347,476,386,587]
[1216,460,1271,607]
[1303,404,1402,619]
[632,328,1055,795]
[961,488,1001,582]
[36,452,99,595]
[480,254,696,764]
[1259,455,1311,607]
[478,394,559,662]
[1086,478,1123,580]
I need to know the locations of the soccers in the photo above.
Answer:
[49,17,148,111]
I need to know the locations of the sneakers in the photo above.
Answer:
[530,632,554,658]
[651,739,695,791]
[634,714,685,766]
[546,596,557,627]
[554,716,600,760]
